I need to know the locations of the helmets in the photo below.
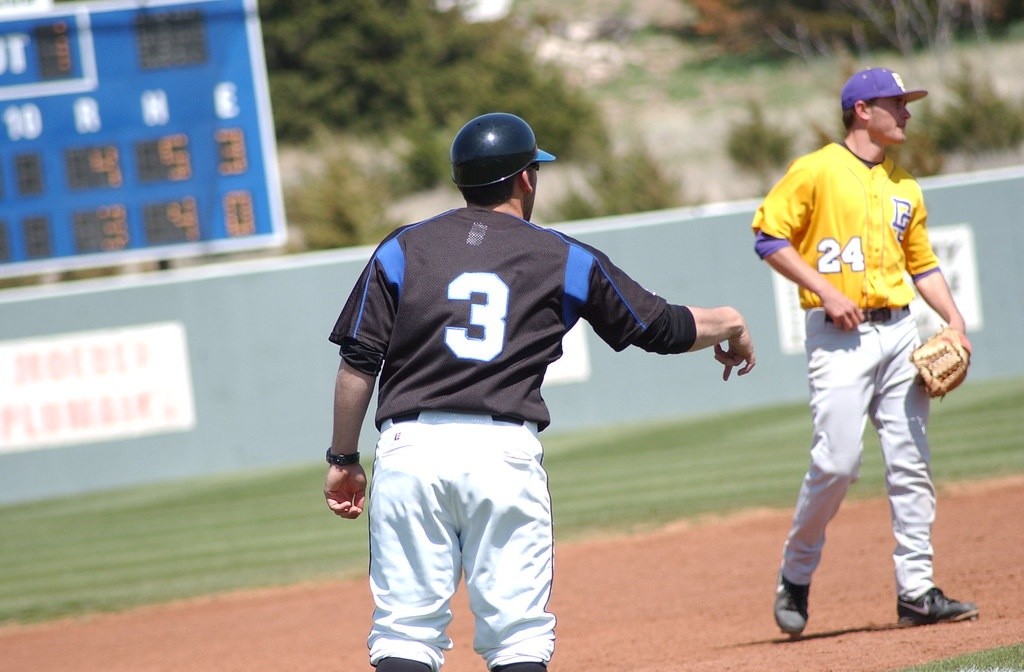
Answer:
[450,112,556,187]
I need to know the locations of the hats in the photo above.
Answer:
[841,66,928,111]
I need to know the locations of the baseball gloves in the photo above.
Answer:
[910,328,973,401]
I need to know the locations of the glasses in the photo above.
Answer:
[527,162,540,171]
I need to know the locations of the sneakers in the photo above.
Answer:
[897,588,979,627]
[775,570,810,634]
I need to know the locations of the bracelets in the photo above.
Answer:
[326,447,360,466]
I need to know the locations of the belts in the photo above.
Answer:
[825,306,909,324]
[393,411,524,425]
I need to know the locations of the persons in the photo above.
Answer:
[751,67,979,635]
[322,114,759,672]
